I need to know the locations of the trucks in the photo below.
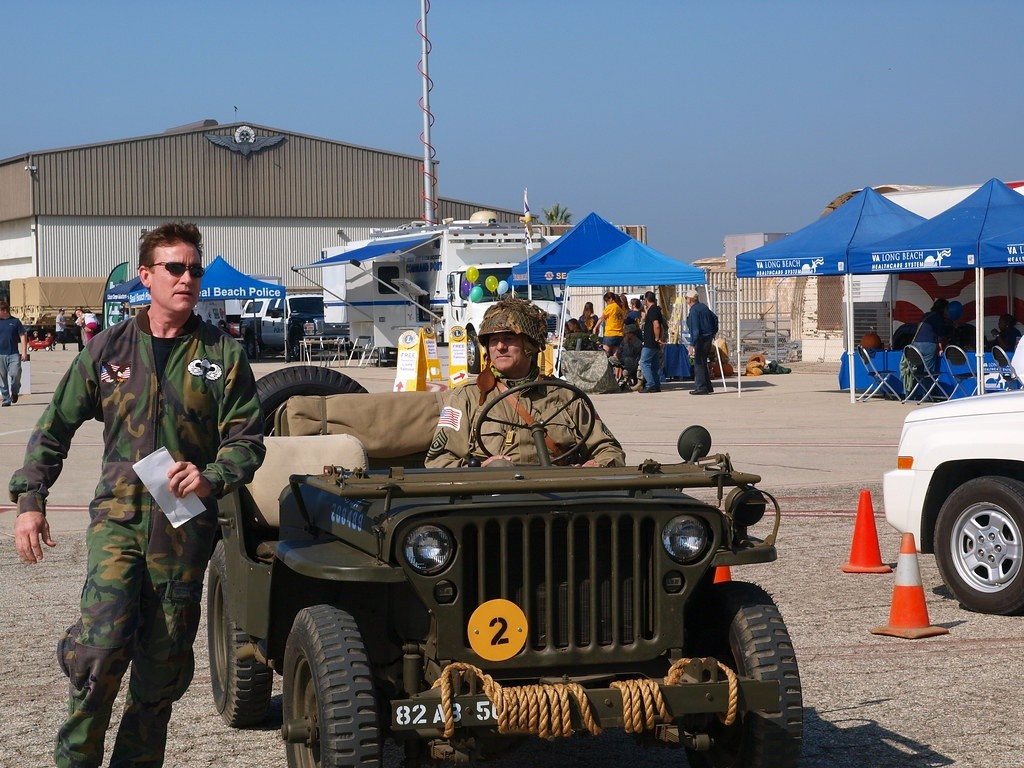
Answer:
[289,215,570,366]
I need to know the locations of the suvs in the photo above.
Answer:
[207,362,807,768]
[239,293,323,360]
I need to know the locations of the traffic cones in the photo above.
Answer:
[712,565,732,583]
[864,533,949,642]
[840,490,892,576]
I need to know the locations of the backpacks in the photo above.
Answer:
[708,309,718,340]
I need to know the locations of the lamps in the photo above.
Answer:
[337,230,342,234]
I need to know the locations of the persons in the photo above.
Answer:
[32,331,41,342]
[563,291,664,394]
[892,298,991,401]
[424,298,627,468]
[45,333,54,351]
[0,301,27,406]
[54,308,68,350]
[73,309,101,352]
[10,218,265,768]
[685,289,715,394]
[986,314,1022,351]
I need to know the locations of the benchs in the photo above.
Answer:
[267,385,445,459]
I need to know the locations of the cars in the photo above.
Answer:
[870,389,1023,615]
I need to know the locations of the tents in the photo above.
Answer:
[508,213,634,300]
[130,256,288,363]
[105,276,146,302]
[555,238,727,392]
[835,178,1024,405]
[735,186,929,399]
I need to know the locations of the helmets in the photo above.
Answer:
[478,297,547,353]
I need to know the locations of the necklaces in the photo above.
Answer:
[496,386,520,446]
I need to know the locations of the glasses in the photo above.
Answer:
[685,297,687,299]
[151,261,204,277]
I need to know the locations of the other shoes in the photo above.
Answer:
[11,393,18,403]
[630,382,643,390]
[52,344,56,351]
[639,386,661,392]
[2,403,11,406]
[690,389,708,394]
[63,348,69,351]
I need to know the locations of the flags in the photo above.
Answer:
[524,190,533,249]
[101,262,130,331]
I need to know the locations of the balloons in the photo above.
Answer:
[496,281,509,294]
[462,267,483,303]
[486,276,498,291]
[946,301,963,320]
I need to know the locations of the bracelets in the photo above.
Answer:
[996,335,1001,337]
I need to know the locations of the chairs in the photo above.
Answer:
[242,434,367,564]
[991,346,1024,391]
[302,335,376,368]
[943,345,981,401]
[856,345,901,402]
[901,345,949,405]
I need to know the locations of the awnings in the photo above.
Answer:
[293,232,441,270]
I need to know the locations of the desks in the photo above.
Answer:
[299,340,346,366]
[838,347,1023,400]
[663,343,691,381]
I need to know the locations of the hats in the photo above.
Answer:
[59,308,66,311]
[686,290,699,300]
